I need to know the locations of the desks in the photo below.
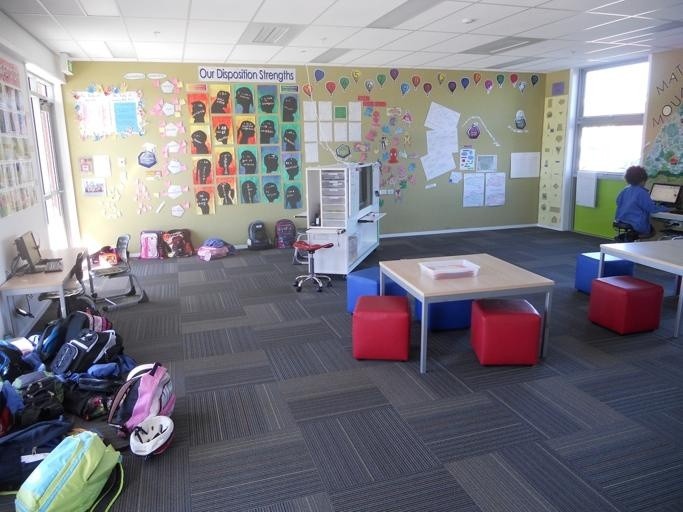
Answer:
[649,210,683,234]
[1,248,94,337]
[378,253,556,374]
[598,240,683,339]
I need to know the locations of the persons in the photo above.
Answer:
[191,87,301,214]
[615,166,676,241]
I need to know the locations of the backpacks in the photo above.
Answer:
[274,218,297,249]
[0,421,74,511]
[1,310,137,436]
[13,431,124,512]
[247,220,269,250]
[197,238,235,262]
[107,361,177,439]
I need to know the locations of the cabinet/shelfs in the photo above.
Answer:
[294,162,387,276]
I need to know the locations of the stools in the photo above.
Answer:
[415,292,471,331]
[574,251,634,296]
[588,276,664,335]
[613,219,634,241]
[471,298,542,368]
[346,267,405,317]
[352,294,412,362]
[293,239,334,293]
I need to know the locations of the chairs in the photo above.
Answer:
[90,234,149,312]
[37,252,94,325]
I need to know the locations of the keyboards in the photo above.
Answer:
[45,261,62,271]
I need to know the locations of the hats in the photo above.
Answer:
[129,415,174,457]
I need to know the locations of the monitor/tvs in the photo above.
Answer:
[15,231,42,272]
[650,183,681,203]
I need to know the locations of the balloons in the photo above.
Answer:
[303,69,538,96]
